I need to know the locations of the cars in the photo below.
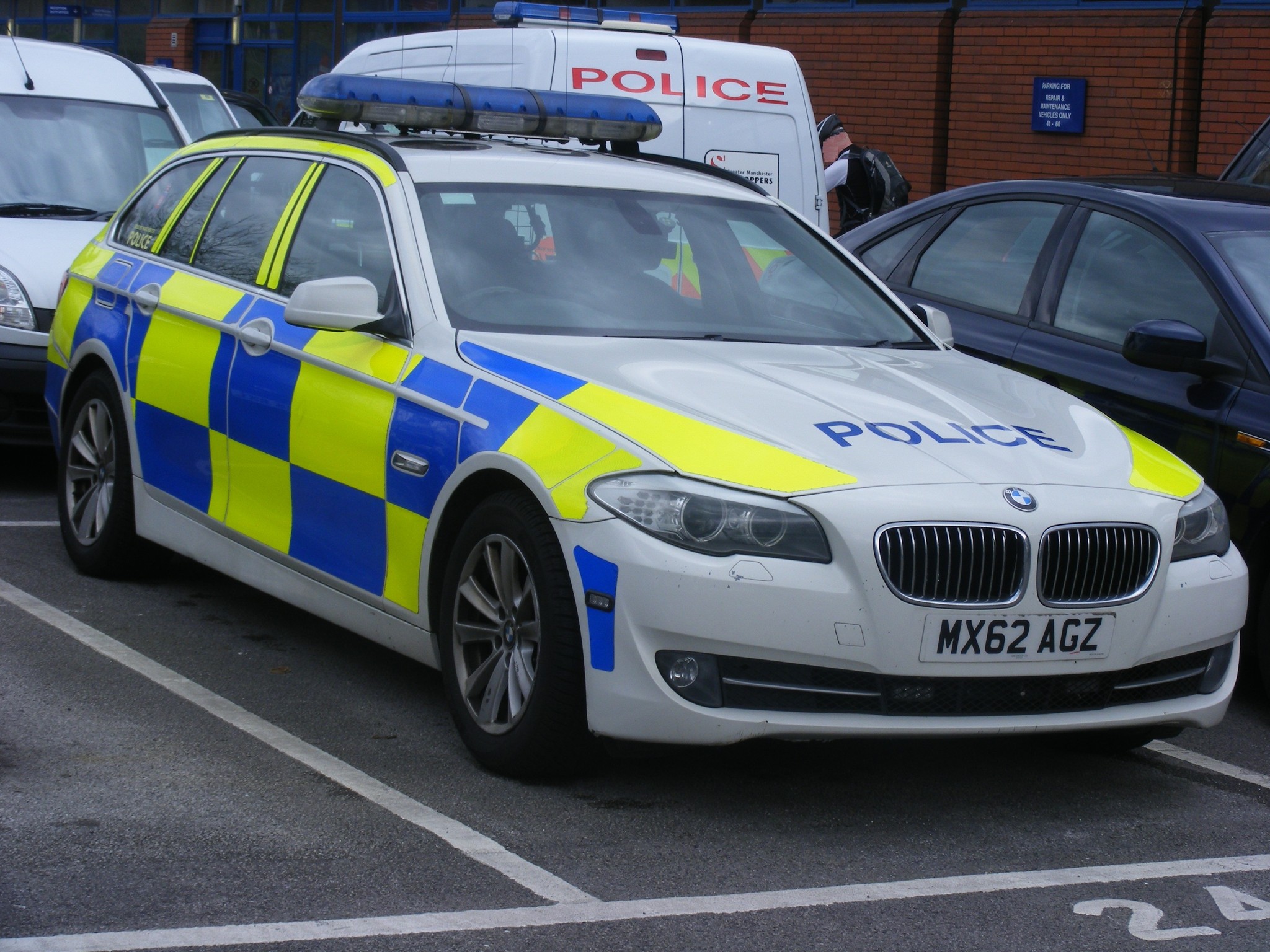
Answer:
[758,178,1270,701]
[37,72,1248,783]
[1218,116,1270,191]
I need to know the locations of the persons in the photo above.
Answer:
[815,114,912,239]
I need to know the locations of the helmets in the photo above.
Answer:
[817,113,844,144]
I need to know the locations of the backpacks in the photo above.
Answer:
[839,144,912,220]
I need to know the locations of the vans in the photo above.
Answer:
[285,1,832,312]
[0,34,218,468]
[136,62,284,196]
